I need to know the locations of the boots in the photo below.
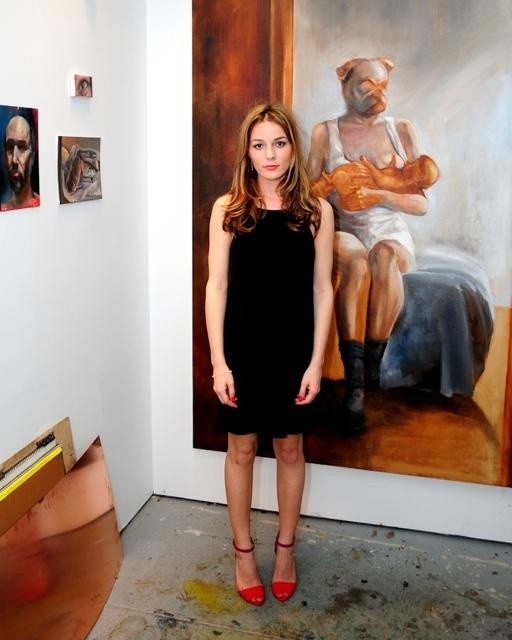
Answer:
[339,336,388,414]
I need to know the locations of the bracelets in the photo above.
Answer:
[212,370,232,378]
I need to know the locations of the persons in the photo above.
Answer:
[203,104,335,607]
[306,56,429,413]
[76,76,92,96]
[0,111,40,212]
[310,155,440,210]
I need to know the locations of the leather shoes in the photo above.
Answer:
[233,538,265,607]
[271,531,298,603]
